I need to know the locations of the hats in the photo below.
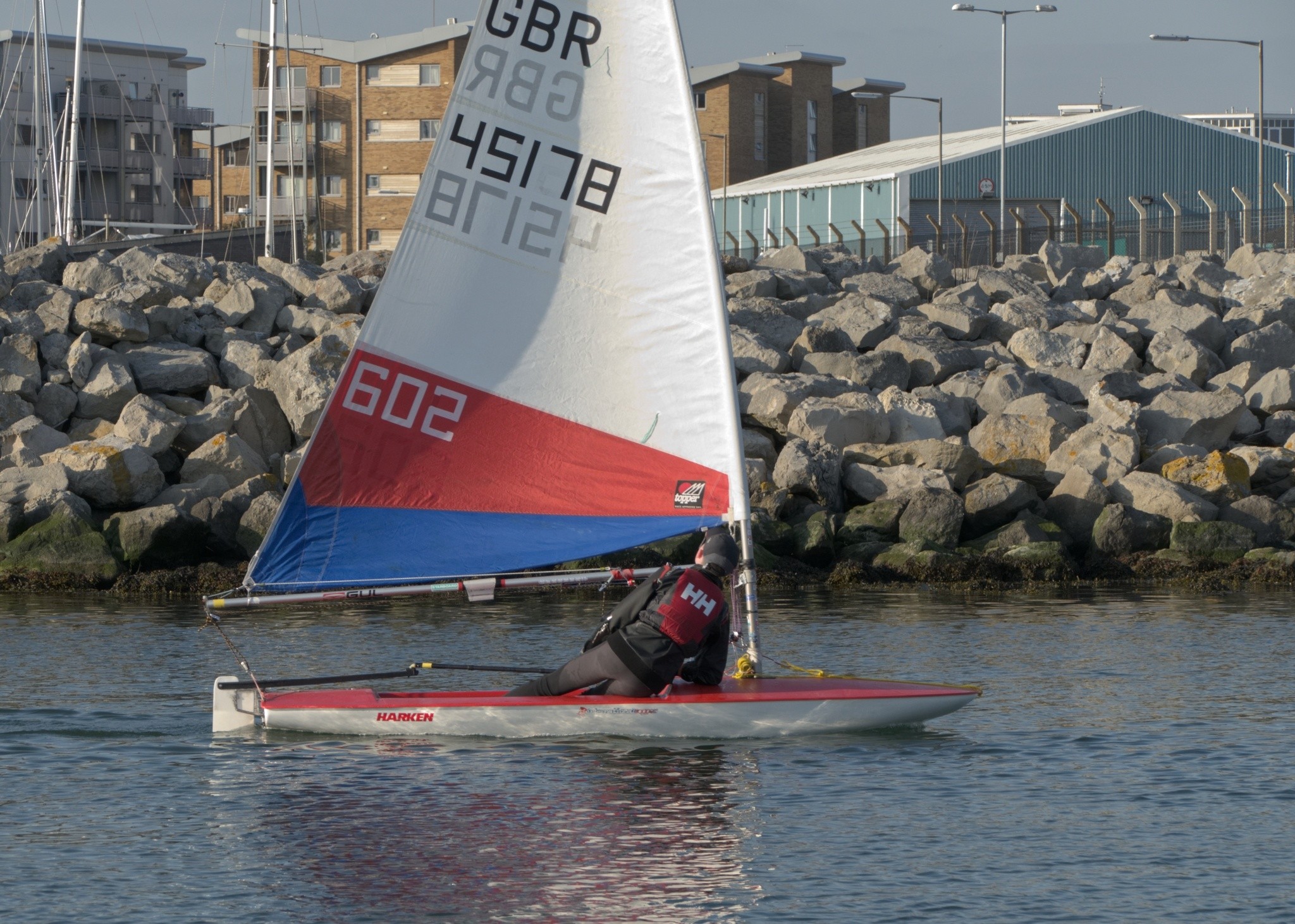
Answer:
[703,527,740,575]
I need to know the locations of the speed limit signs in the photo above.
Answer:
[979,178,994,193]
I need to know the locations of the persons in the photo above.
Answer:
[503,527,739,698]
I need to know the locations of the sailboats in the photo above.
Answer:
[0,0,325,266]
[198,0,988,741]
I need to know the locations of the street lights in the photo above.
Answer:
[951,3,1059,264]
[849,92,944,230]
[1148,33,1265,252]
